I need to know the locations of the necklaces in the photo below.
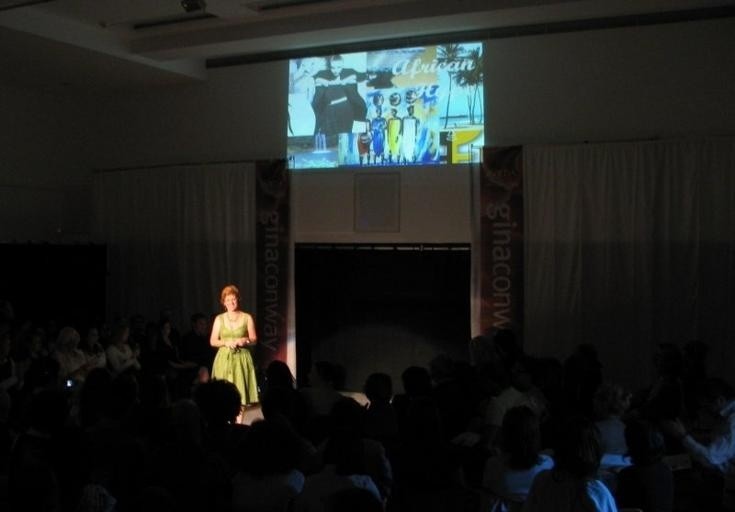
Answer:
[226,311,241,322]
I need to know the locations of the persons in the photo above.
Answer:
[209,285,260,425]
[0,312,735,511]
[310,55,420,165]
